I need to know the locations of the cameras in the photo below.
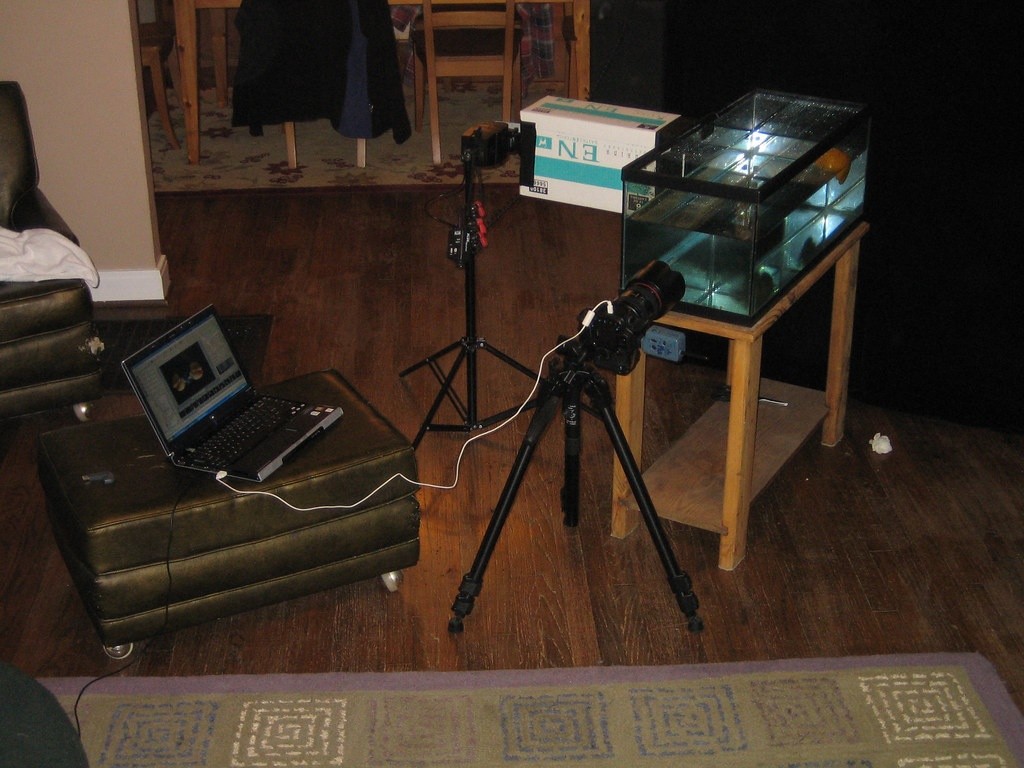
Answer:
[578,259,685,376]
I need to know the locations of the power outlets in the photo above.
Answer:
[639,324,686,363]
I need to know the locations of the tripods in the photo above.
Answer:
[449,335,703,632]
[399,151,604,452]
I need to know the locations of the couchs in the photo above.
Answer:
[0,81,102,423]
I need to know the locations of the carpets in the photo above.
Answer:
[28,652,1024,768]
[90,314,275,397]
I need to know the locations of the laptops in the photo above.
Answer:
[121,303,344,483]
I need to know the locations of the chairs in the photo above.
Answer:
[412,1,521,166]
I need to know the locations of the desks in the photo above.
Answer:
[174,0,590,167]
[608,223,872,570]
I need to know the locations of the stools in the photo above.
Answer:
[35,368,422,661]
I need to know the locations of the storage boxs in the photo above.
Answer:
[619,88,872,330]
[516,96,682,219]
